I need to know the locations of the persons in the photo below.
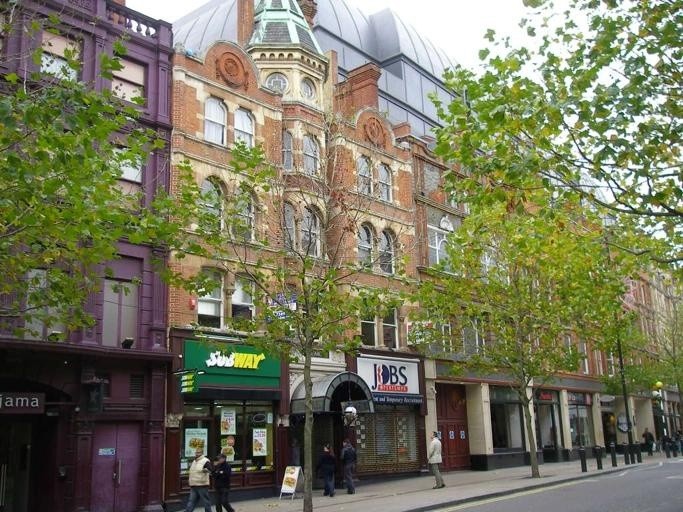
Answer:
[184,446,212,511]
[290,435,300,467]
[427,430,445,490]
[641,427,654,456]
[654,428,682,453]
[319,441,336,496]
[211,454,234,511]
[338,437,355,495]
